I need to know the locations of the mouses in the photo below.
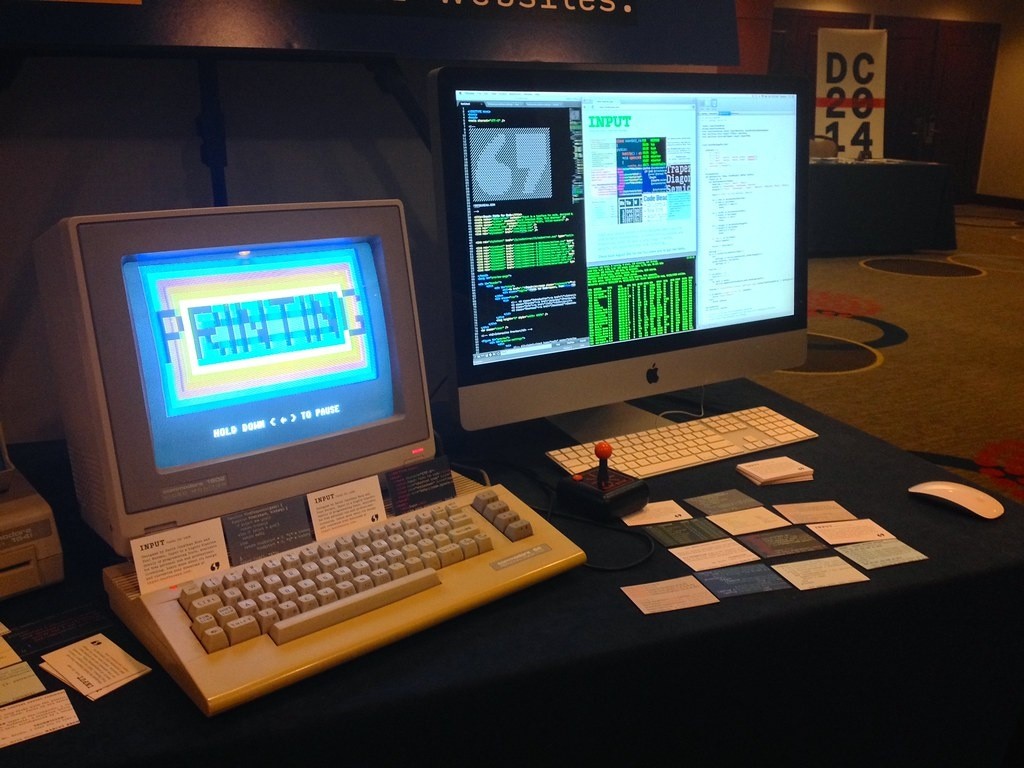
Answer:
[908,481,1004,519]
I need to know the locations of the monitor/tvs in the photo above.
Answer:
[429,60,808,445]
[32,198,438,557]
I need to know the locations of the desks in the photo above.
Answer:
[0,380,1024,768]
[805,156,959,259]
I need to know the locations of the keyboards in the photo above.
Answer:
[135,482,587,721]
[543,406,819,479]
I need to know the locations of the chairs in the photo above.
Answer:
[808,136,838,158]
